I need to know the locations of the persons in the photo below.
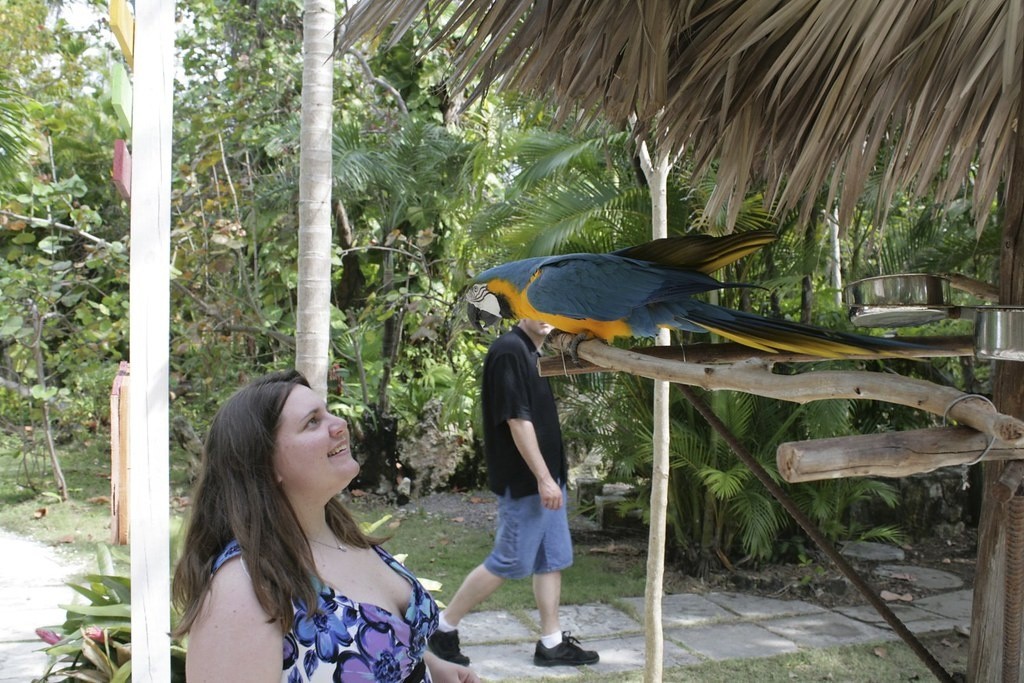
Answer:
[169,369,487,683]
[428,319,600,667]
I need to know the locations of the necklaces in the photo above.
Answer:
[306,537,347,553]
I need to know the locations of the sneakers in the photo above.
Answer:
[426,628,470,667]
[533,630,600,666]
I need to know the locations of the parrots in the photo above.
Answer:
[463,226,961,373]
